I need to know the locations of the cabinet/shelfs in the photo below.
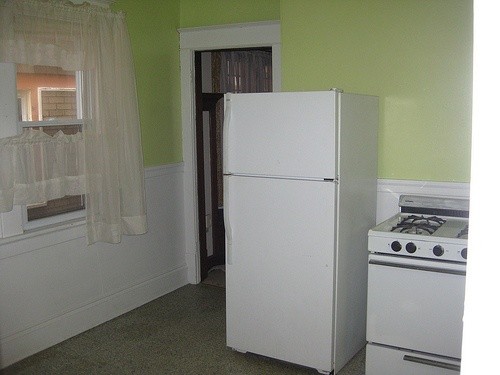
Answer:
[365,255,468,375]
